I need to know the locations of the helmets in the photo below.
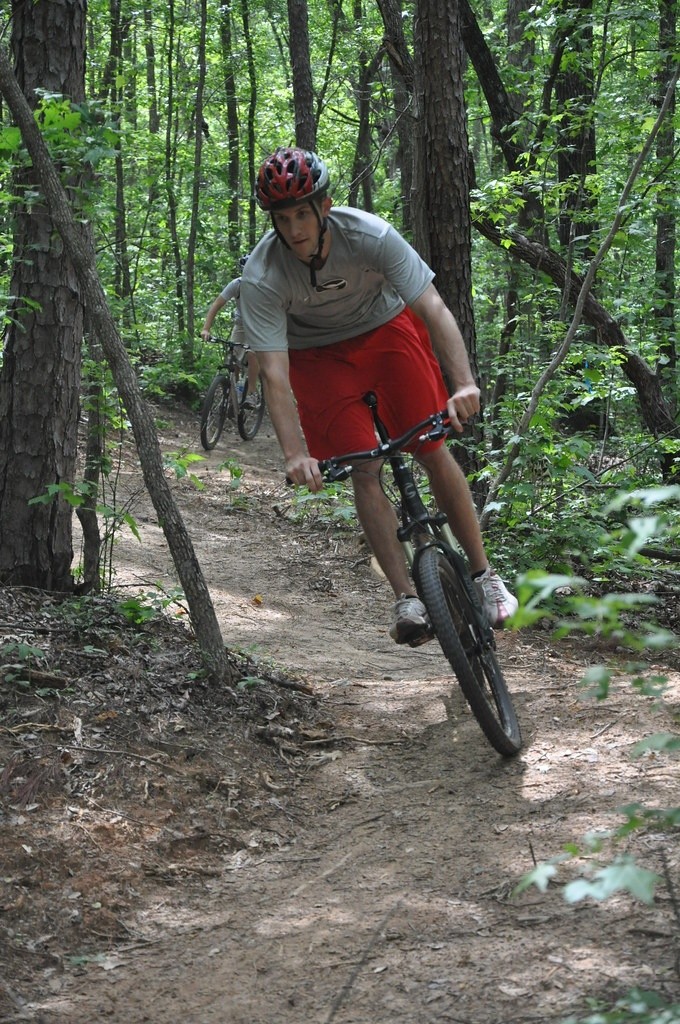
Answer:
[239,253,251,270]
[253,146,331,211]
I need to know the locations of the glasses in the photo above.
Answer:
[239,259,246,265]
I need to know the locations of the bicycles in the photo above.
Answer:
[283,389,524,759]
[193,332,268,451]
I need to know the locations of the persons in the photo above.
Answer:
[241,146,518,648]
[201,254,261,415]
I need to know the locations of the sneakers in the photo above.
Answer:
[388,592,433,644]
[242,391,261,410]
[472,566,519,629]
[218,391,233,414]
